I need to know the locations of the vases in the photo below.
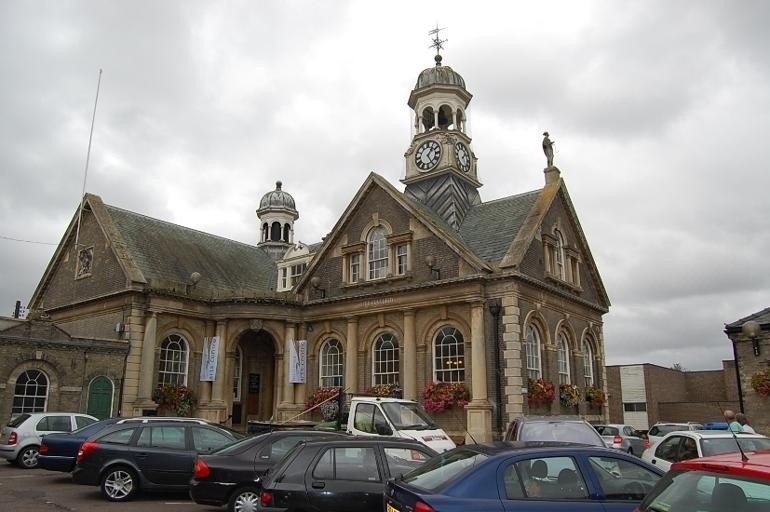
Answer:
[563,393,570,401]
[589,391,594,399]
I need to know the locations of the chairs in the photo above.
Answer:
[531,461,548,490]
[558,469,578,497]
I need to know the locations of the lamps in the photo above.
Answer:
[425,254,439,272]
[311,276,325,292]
[742,321,761,356]
[188,272,201,286]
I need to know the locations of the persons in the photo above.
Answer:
[736,412,756,433]
[672,436,704,463]
[724,410,744,432]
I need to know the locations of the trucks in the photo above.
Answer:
[248,397,457,463]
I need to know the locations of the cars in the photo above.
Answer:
[385,441,667,512]
[505,416,620,484]
[259,437,440,512]
[594,420,770,510]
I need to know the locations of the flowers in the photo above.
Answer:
[527,377,556,401]
[422,379,470,413]
[307,385,343,411]
[560,383,581,407]
[153,383,196,417]
[367,383,401,399]
[750,369,770,395]
[590,387,607,409]
